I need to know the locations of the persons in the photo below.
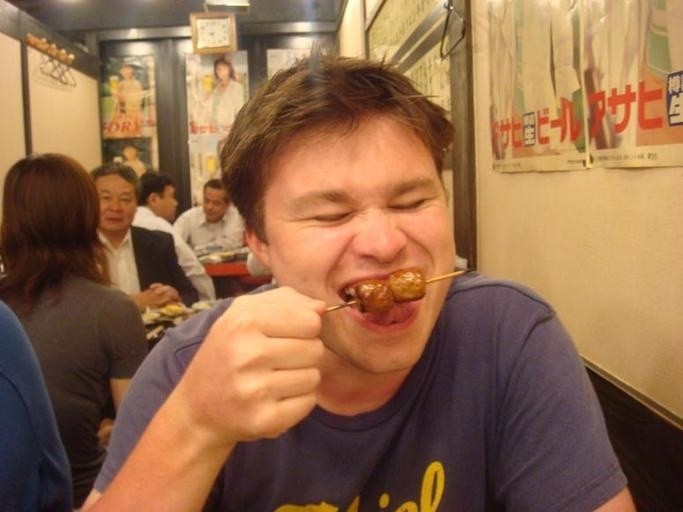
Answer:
[80,52,636,512]
[112,61,144,136]
[199,58,245,150]
[90,162,199,313]
[129,172,215,299]
[173,179,245,298]
[2,153,150,510]
[212,139,227,179]
[1,302,73,511]
[119,140,147,176]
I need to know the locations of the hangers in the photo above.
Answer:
[439,1,471,60]
[38,42,77,87]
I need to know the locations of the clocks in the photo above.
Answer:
[190,11,237,55]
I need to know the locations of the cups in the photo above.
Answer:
[203,71,212,93]
[207,155,216,176]
[109,75,119,94]
